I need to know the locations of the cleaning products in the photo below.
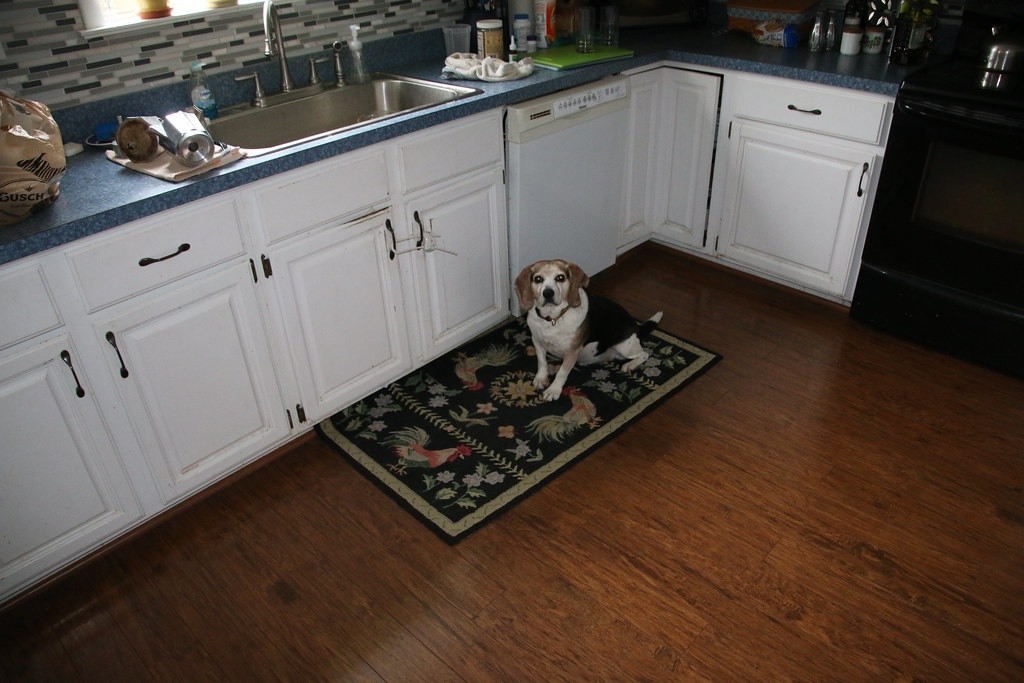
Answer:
[190,59,219,121]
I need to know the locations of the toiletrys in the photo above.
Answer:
[344,24,372,85]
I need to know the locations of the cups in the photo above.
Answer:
[598,6,619,49]
[442,24,471,56]
[575,6,596,53]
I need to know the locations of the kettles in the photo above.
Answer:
[984,25,1024,73]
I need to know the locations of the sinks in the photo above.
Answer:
[203,71,486,159]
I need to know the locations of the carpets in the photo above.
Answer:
[314,313,726,548]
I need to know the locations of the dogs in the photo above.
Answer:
[515,259,663,402]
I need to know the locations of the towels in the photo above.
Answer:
[439,51,535,82]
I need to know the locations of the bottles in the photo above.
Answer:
[527,34,537,54]
[808,11,824,53]
[751,20,800,49]
[534,1,556,48]
[509,35,518,63]
[513,14,532,51]
[476,19,503,61]
[840,17,862,55]
[825,10,836,53]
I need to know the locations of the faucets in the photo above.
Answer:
[263,0,293,92]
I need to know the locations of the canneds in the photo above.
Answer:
[476,19,504,62]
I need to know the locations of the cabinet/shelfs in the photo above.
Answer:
[52,187,300,524]
[0,249,156,611]
[238,105,512,434]
[703,70,896,325]
